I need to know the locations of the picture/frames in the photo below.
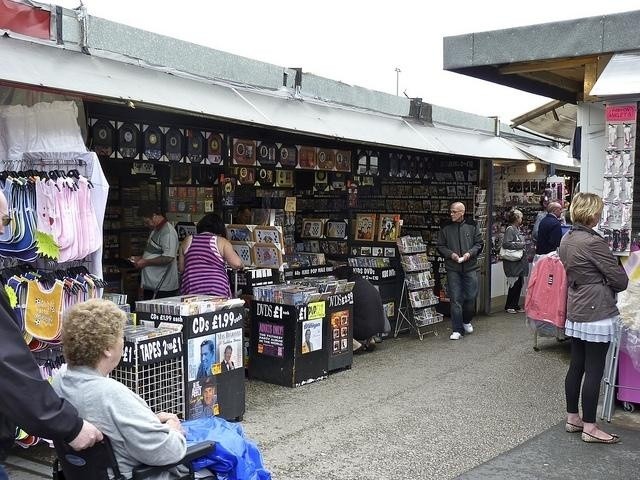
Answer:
[224,223,254,243]
[300,218,325,239]
[354,213,378,243]
[227,240,253,268]
[173,219,200,242]
[252,241,284,271]
[323,218,349,241]
[251,224,286,257]
[376,213,403,243]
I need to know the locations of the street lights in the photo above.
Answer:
[394,67,401,96]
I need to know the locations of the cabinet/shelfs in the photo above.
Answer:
[349,262,412,341]
[283,260,335,282]
[135,304,248,424]
[245,299,329,389]
[325,288,357,376]
[391,232,447,342]
[489,258,508,300]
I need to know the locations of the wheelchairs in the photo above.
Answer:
[52,427,217,480]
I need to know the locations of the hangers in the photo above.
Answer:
[0,261,112,297]
[1,156,96,196]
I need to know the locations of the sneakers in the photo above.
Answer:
[582,432,621,443]
[450,331,464,339]
[565,422,584,432]
[463,324,473,333]
[506,308,516,313]
[517,309,526,312]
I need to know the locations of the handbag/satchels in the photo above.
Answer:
[500,242,523,261]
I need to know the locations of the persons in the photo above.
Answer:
[502,209,529,314]
[194,378,216,413]
[304,328,313,354]
[177,211,243,296]
[304,223,311,237]
[0,191,105,479]
[535,201,562,254]
[129,200,180,299]
[558,192,628,445]
[364,226,372,239]
[221,345,236,372]
[50,297,272,479]
[331,264,386,355]
[329,223,333,236]
[196,340,215,379]
[531,195,551,243]
[437,201,484,340]
[357,226,365,238]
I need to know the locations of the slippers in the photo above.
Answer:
[354,342,363,354]
[366,340,376,350]
